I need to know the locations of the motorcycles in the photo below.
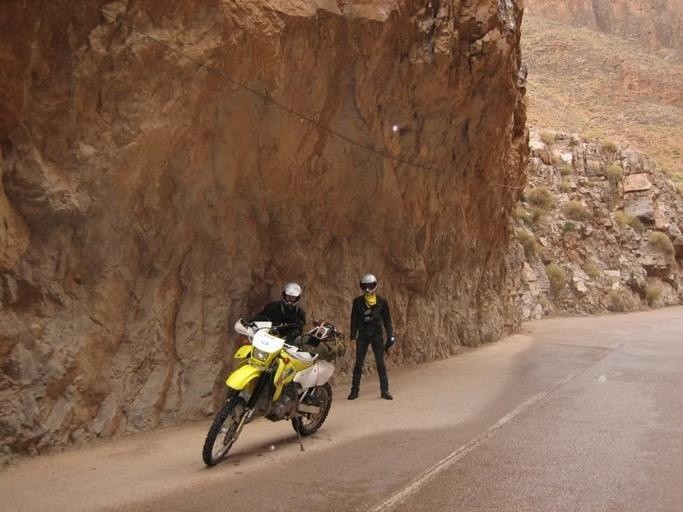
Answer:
[202,315,347,467]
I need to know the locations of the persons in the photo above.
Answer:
[346,273,395,401]
[241,281,306,346]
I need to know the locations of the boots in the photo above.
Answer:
[381,388,393,400]
[347,387,359,400]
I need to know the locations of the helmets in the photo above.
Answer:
[358,271,378,295]
[280,282,302,307]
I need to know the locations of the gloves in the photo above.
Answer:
[385,337,396,354]
